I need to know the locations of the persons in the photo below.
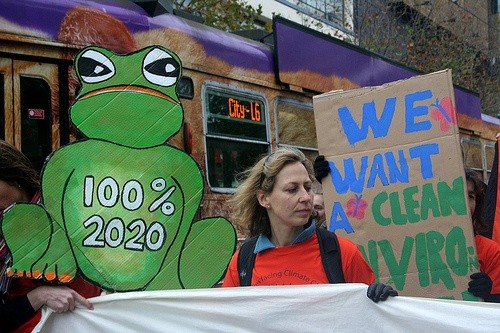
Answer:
[0,140,95,333]
[9,134,500,333]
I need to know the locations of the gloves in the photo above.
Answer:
[467,273,492,299]
[367,282,398,304]
[313,155,331,184]
